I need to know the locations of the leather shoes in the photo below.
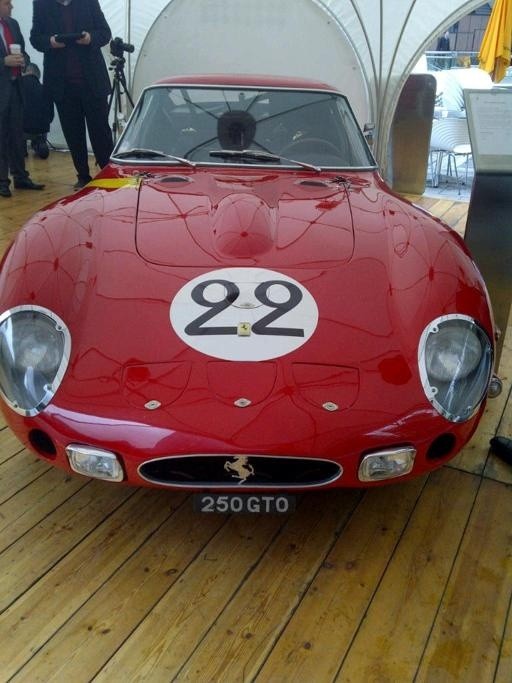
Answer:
[13,181,46,189]
[0,185,11,197]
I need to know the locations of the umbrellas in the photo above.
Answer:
[477,0,512,83]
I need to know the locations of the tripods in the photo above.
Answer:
[95,57,134,166]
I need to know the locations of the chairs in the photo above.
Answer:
[426,70,473,195]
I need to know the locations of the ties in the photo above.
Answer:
[1,20,21,76]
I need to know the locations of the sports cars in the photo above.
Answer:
[0,72,503,518]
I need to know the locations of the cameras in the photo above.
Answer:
[110,37,134,58]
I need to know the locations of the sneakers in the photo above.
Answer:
[72,179,84,190]
[37,135,48,158]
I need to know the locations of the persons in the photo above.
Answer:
[29,0,114,190]
[0,0,45,197]
[436,31,450,69]
[22,63,54,159]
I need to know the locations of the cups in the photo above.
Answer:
[9,43,22,54]
